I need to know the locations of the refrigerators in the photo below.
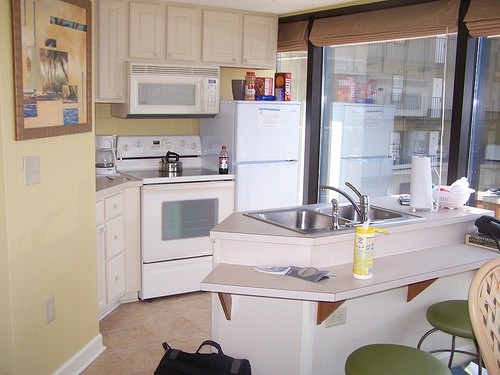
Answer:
[327,101,396,204]
[200,100,301,213]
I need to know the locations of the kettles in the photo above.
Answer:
[159,151,182,172]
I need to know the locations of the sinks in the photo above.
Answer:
[314,204,404,221]
[242,208,351,234]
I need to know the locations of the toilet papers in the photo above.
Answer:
[409,155,434,213]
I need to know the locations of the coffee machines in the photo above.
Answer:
[95,136,120,176]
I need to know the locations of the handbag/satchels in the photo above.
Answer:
[153,339,252,375]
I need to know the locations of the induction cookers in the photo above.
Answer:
[115,135,236,185]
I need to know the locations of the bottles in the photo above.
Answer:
[244,71,256,100]
[218,145,229,175]
[366,79,378,103]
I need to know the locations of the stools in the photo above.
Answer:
[345,343,454,375]
[417,299,482,375]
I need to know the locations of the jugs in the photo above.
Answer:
[96,148,122,169]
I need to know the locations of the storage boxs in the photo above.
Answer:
[356,98,376,103]
[275,72,292,101]
[255,77,273,96]
[355,82,372,100]
[275,87,285,101]
[255,95,276,101]
[335,75,357,103]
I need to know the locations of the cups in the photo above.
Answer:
[231,80,245,100]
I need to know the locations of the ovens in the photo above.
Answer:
[138,181,236,301]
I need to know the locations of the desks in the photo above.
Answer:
[200,262,345,327]
[332,240,500,303]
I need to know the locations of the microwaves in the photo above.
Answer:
[125,61,220,116]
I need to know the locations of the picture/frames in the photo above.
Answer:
[11,0,92,142]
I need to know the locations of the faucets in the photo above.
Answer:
[318,181,386,227]
[329,198,340,231]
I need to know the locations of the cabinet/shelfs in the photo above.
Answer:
[383,36,438,82]
[95,186,141,320]
[129,0,202,66]
[92,0,130,104]
[202,5,279,70]
[332,41,383,79]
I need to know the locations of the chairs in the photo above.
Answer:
[467,255,500,375]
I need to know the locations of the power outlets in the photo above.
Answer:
[324,306,347,329]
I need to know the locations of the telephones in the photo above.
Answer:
[475,215,500,237]
